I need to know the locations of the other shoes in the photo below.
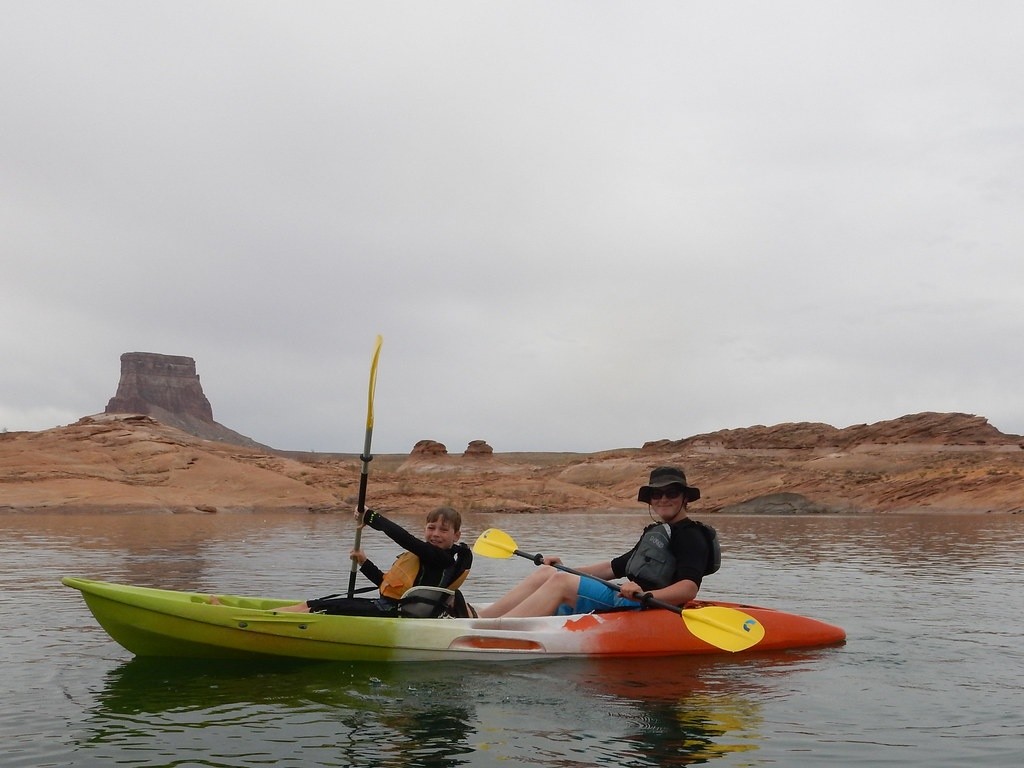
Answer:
[454,588,478,619]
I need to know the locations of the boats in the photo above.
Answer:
[57,572,848,667]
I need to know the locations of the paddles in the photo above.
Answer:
[470,527,766,653]
[347,333,385,598]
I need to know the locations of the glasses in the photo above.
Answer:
[649,488,685,500]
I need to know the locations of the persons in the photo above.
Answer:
[455,464,721,620]
[209,504,464,618]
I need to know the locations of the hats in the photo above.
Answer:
[638,467,700,506]
